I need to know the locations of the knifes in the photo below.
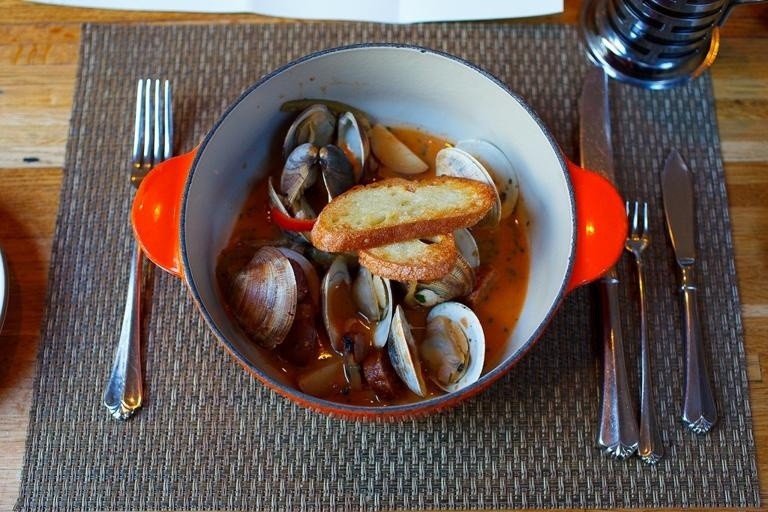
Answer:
[655,145,722,438]
[577,70,644,460]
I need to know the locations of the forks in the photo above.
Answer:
[625,196,667,465]
[93,79,175,427]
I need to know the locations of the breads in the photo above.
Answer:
[308,176,496,281]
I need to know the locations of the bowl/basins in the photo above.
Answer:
[131,40,627,427]
